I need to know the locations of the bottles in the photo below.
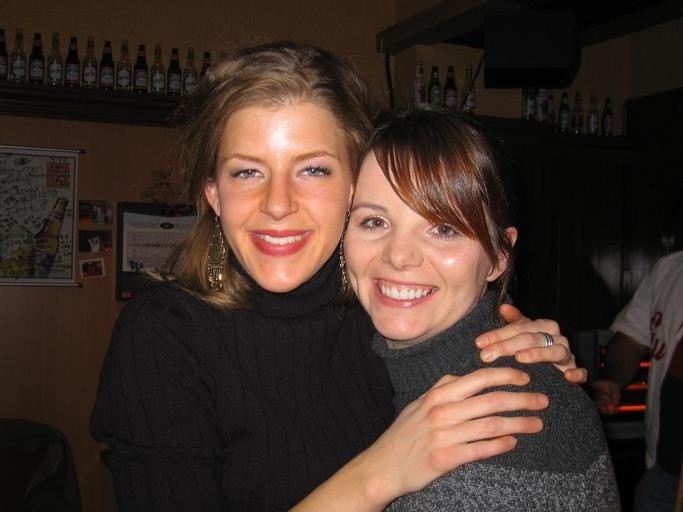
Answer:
[519,84,616,141]
[29,192,69,274]
[0,28,216,97]
[415,60,478,112]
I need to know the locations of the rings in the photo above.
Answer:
[539,331,555,346]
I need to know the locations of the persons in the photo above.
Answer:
[91,41,588,511]
[588,240,683,512]
[343,103,621,511]
